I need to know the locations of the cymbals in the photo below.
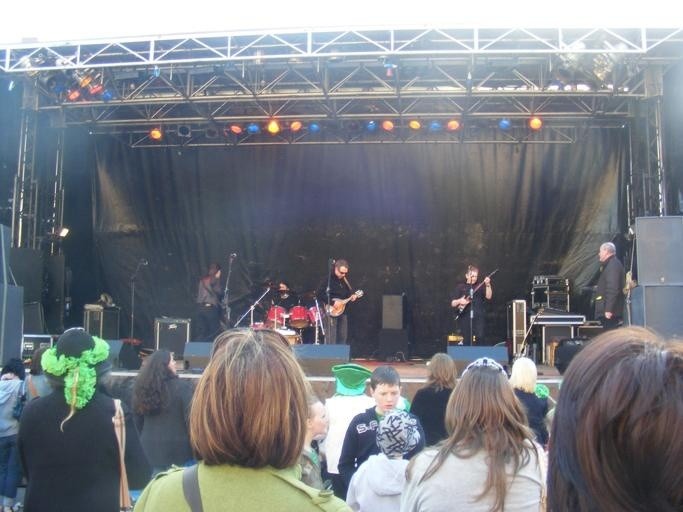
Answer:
[305,291,318,301]
[258,283,275,288]
[278,290,289,293]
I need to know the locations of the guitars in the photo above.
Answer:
[453,268,499,320]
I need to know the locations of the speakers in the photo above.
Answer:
[377,328,409,362]
[554,337,590,374]
[182,341,213,371]
[445,345,509,378]
[105,339,142,371]
[382,294,406,329]
[633,216,683,285]
[622,284,683,343]
[294,344,351,378]
[0,224,65,369]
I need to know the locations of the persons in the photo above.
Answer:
[271,282,299,312]
[452,264,493,347]
[1,327,683,511]
[595,242,626,331]
[319,260,357,345]
[196,263,224,341]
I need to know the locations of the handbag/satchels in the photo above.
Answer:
[11,381,26,421]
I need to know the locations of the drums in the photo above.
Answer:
[268,306,285,324]
[309,306,325,326]
[253,322,263,329]
[289,306,309,328]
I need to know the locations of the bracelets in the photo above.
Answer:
[485,284,490,288]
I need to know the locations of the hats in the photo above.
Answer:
[43,328,113,386]
[375,411,422,457]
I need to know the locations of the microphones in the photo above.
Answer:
[230,253,237,257]
[143,259,148,265]
[468,265,474,270]
[329,259,336,264]
